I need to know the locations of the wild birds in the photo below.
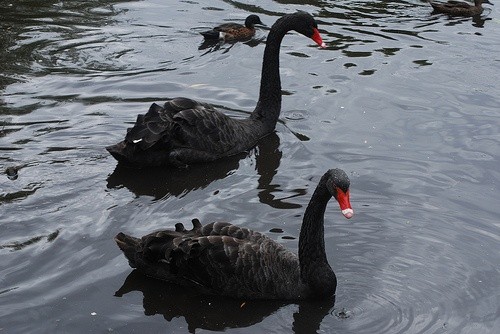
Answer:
[105,12,326,167]
[114,168,354,297]
[198,14,267,39]
[429,0,494,13]
